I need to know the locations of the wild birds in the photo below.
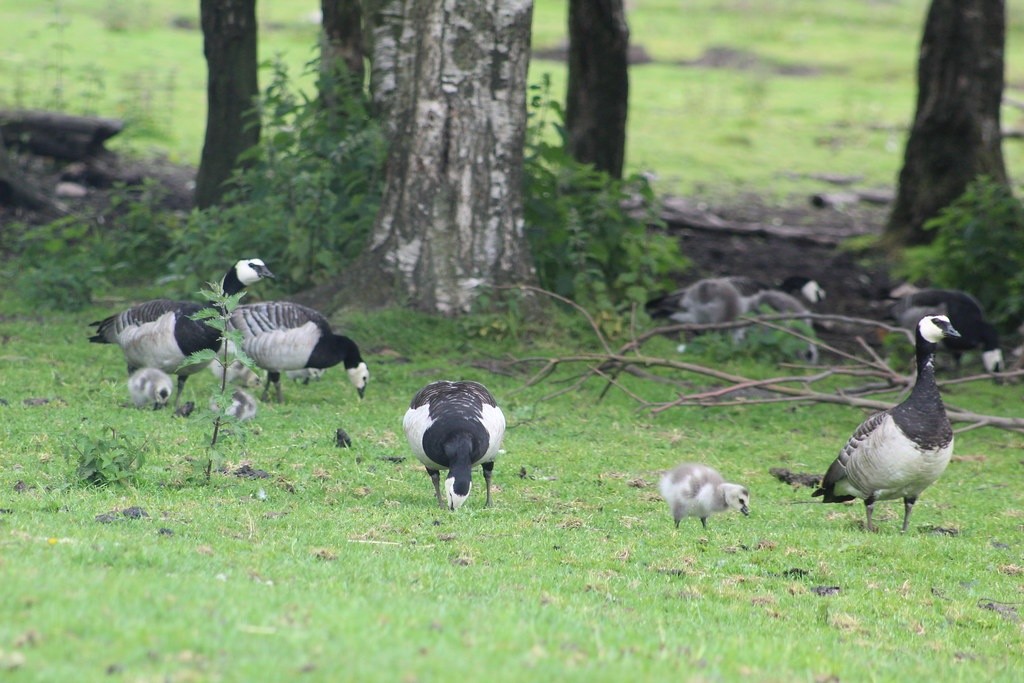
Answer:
[401,379,506,508]
[655,462,749,530]
[87,259,371,426]
[644,269,1007,390]
[809,316,961,535]
